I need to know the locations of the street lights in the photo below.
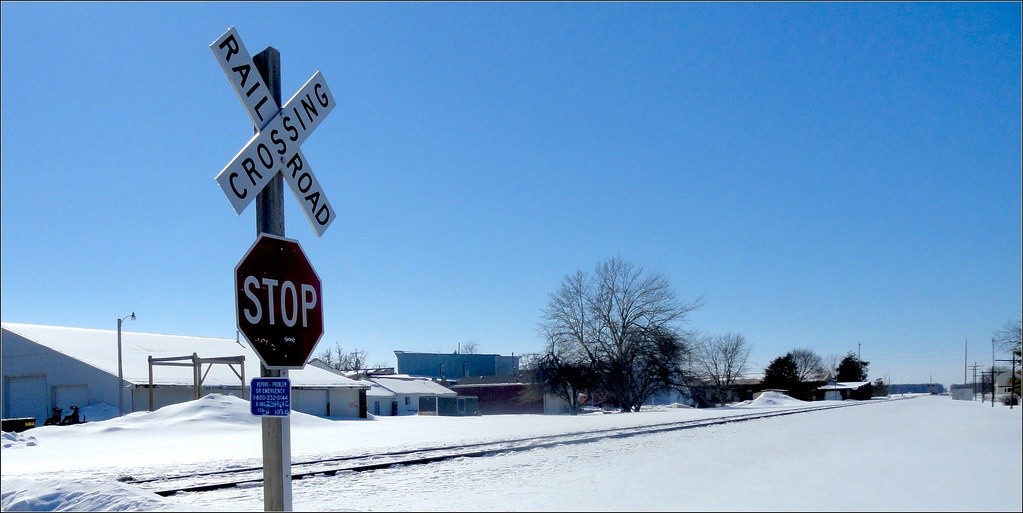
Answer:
[117,311,137,417]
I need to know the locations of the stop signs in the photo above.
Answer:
[231,232,327,371]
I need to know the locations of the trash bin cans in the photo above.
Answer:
[1,417,36,433]
